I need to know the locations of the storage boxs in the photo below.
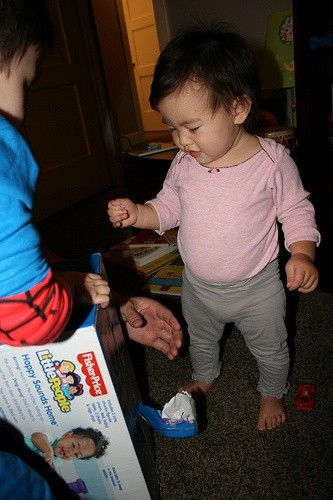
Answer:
[0,251,159,499]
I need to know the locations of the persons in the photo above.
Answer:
[0,0,111,500]
[24,427,113,469]
[106,28,324,431]
[114,289,185,362]
